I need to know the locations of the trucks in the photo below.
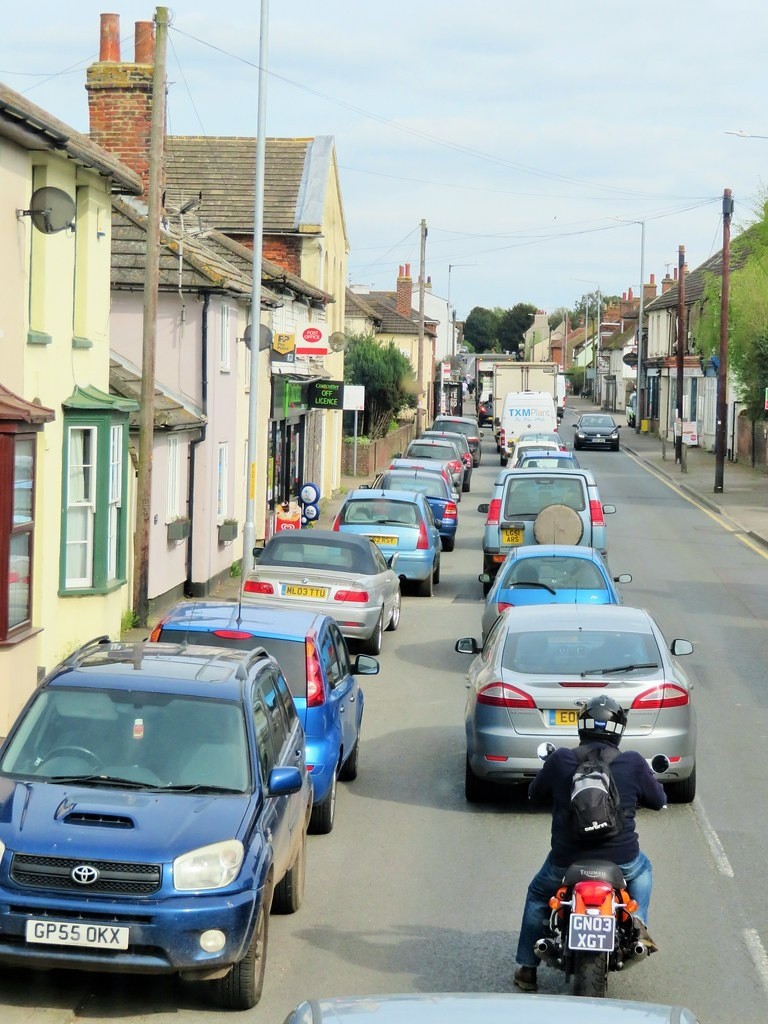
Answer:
[493,361,566,466]
[473,354,517,412]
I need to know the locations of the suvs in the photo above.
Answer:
[0,636,315,1010]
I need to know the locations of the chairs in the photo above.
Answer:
[328,555,347,566]
[282,551,302,562]
[557,459,568,467]
[528,461,537,467]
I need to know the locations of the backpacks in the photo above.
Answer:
[571,747,635,845]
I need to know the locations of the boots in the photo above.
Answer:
[513,966,538,990]
[632,918,658,953]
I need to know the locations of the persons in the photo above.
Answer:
[512,695,667,990]
[462,379,475,402]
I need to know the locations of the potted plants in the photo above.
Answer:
[219,519,239,540]
[168,514,191,540]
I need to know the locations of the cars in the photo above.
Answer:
[479,544,632,646]
[478,468,617,597]
[506,432,580,467]
[359,469,458,552]
[327,489,442,597]
[283,992,701,1024]
[478,401,494,428]
[571,413,622,451]
[455,604,696,804]
[239,529,401,655]
[386,431,473,502]
[626,392,637,428]
[147,599,380,835]
[425,415,484,469]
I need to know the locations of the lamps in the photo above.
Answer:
[179,200,199,213]
[650,351,676,356]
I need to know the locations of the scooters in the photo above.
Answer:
[534,742,670,998]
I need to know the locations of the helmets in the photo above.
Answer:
[578,696,627,749]
